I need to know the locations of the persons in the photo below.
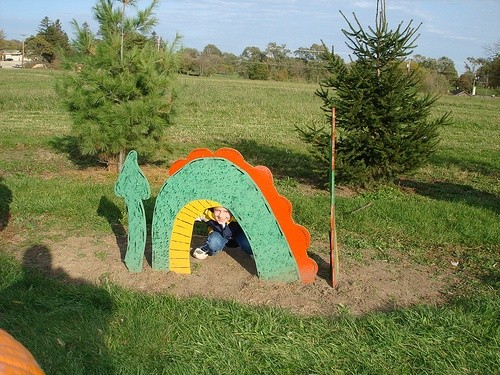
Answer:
[193,206,255,260]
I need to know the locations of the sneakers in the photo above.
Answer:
[194,245,210,260]
